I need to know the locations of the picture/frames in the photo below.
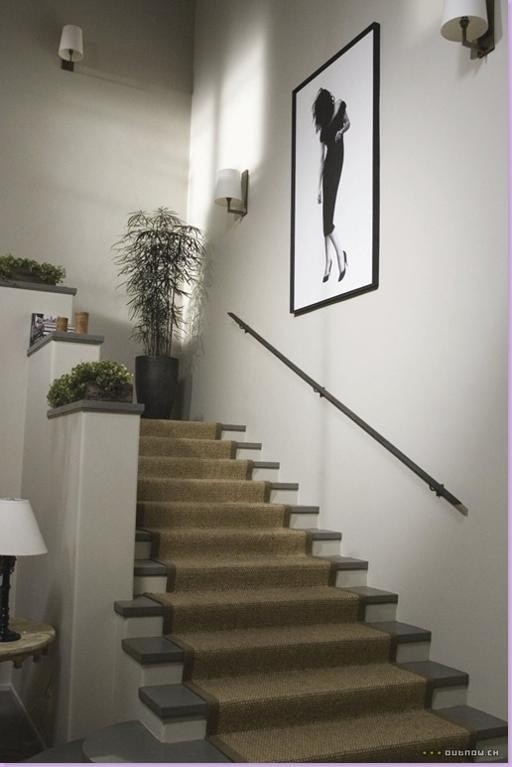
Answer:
[288,21,380,319]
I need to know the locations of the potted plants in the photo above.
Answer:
[108,210,202,419]
[45,356,134,409]
[2,253,67,285]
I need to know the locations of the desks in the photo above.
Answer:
[1,614,56,669]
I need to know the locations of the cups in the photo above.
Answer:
[56,317,69,332]
[75,311,89,335]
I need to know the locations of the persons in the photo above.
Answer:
[308,85,353,284]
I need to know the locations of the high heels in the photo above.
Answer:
[338,250,349,281]
[322,260,332,282]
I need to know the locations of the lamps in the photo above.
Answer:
[213,169,251,222]
[55,23,85,75]
[439,0,497,60]
[0,496,48,642]
